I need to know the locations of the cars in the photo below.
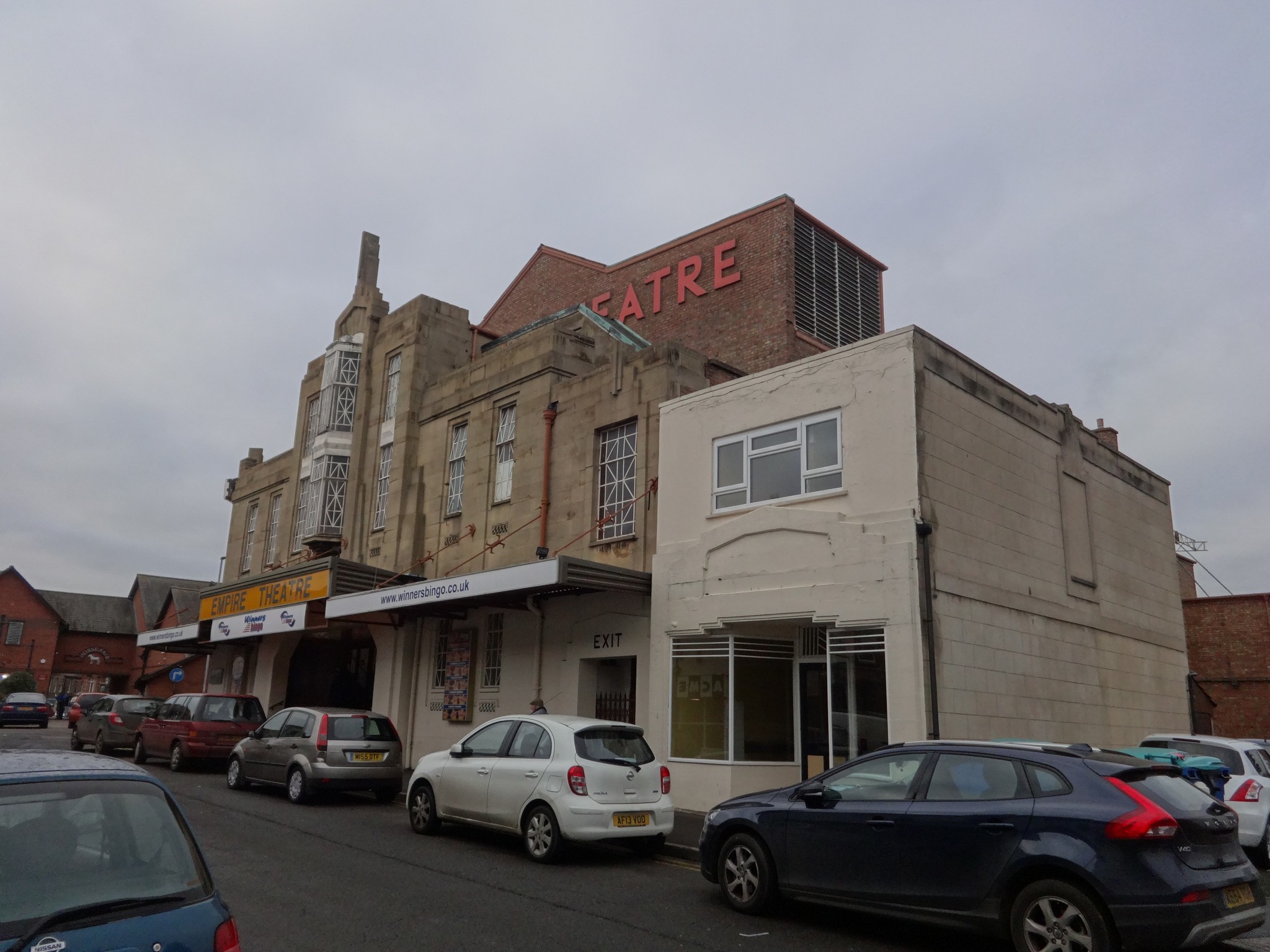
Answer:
[1139,732,1270,866]
[227,706,403,803]
[70,694,166,754]
[406,713,673,861]
[1,748,241,952]
[68,693,110,728]
[1,693,49,729]
[699,738,1267,951]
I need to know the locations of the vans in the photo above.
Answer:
[132,692,267,772]
